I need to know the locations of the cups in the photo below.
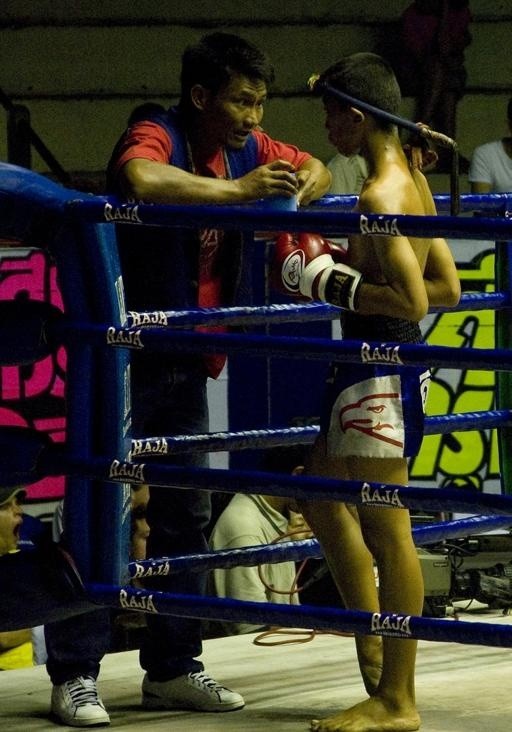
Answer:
[264,168,298,211]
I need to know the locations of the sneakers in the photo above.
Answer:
[139,671,245,712]
[49,675,112,727]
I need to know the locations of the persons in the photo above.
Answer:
[0,478,64,673]
[44,33,332,727]
[402,2,476,177]
[317,148,368,253]
[204,417,322,635]
[107,483,151,654]
[466,98,511,222]
[272,49,461,730]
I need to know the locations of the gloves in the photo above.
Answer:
[273,228,362,312]
